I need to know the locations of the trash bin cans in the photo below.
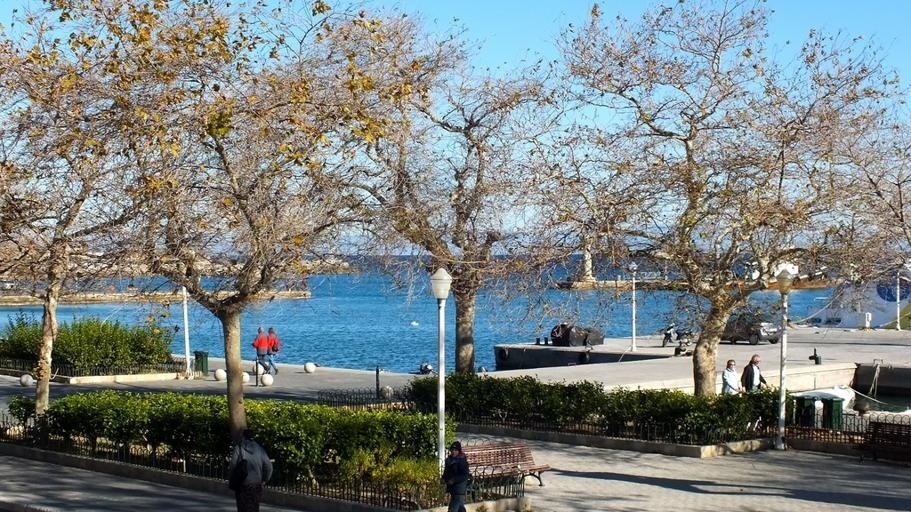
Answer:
[792,391,845,431]
[193,351,209,376]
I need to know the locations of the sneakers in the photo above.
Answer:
[266,368,270,374]
[274,369,278,375]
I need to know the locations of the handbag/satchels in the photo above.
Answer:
[272,344,277,352]
[228,441,248,492]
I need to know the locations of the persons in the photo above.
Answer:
[722,359,742,395]
[741,354,769,392]
[225,428,273,511]
[440,440,470,512]
[251,327,270,374]
[265,327,280,375]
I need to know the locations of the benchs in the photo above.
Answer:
[445,438,551,493]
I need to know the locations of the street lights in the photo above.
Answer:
[628,262,638,352]
[429,268,452,477]
[772,260,799,450]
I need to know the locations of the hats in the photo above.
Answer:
[450,442,461,450]
